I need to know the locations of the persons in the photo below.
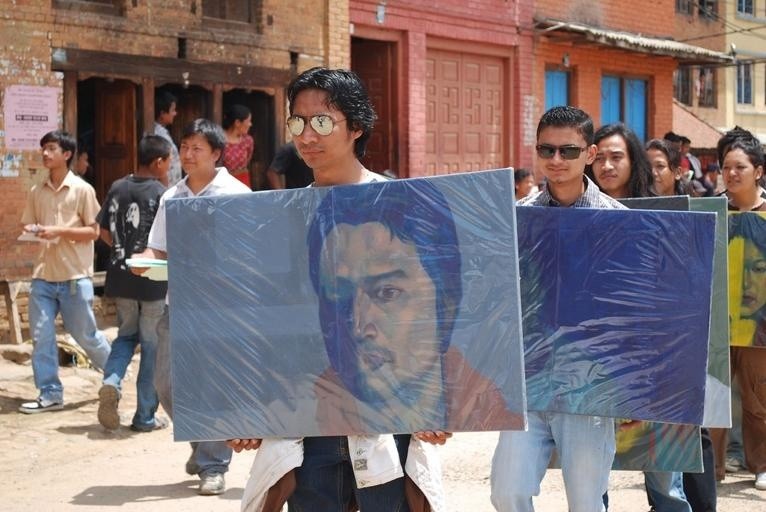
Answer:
[489,108,628,511]
[215,103,253,190]
[583,124,691,510]
[150,89,182,189]
[289,181,524,440]
[515,168,535,202]
[18,131,113,413]
[129,118,256,496]
[93,135,173,431]
[226,66,452,512]
[640,126,766,512]
[266,140,315,191]
[76,146,89,178]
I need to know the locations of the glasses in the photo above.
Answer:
[536,144,586,159]
[287,115,346,136]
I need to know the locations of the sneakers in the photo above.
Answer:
[98,384,120,429]
[19,395,64,413]
[199,473,226,495]
[137,418,168,430]
[754,473,766,489]
[726,458,742,472]
